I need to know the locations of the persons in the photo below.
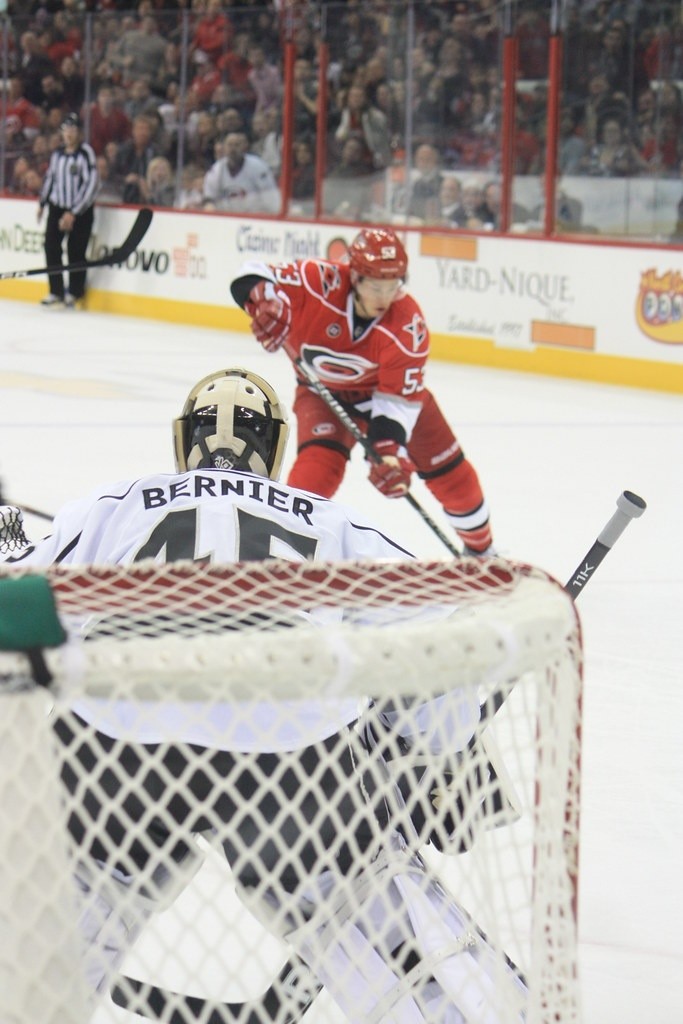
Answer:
[532,167,583,229]
[229,230,493,560]
[246,47,282,137]
[36,113,97,308]
[201,130,281,216]
[0,368,531,1024]
[0,0,683,245]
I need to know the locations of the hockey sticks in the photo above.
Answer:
[1,206,153,279]
[281,338,464,564]
[109,488,647,1024]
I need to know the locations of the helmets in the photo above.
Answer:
[59,113,82,136]
[172,369,290,481]
[344,227,408,285]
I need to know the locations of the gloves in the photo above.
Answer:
[230,280,294,352]
[364,439,419,499]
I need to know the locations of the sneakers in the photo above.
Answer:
[64,288,86,307]
[41,293,65,305]
